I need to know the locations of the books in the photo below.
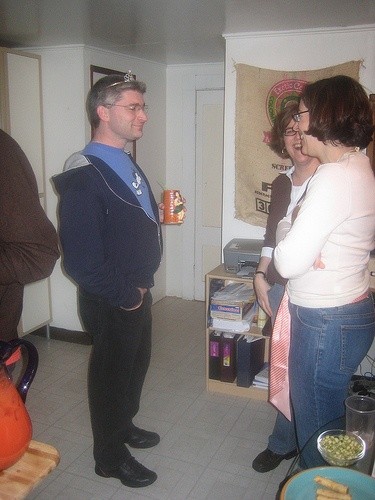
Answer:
[208,329,269,390]
[210,279,258,333]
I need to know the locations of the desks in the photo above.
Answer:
[276,409,375,500]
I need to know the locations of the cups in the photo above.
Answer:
[163,190,184,225]
[345,396,375,448]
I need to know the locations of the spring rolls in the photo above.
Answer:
[314,477,352,500]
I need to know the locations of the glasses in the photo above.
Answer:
[281,127,301,137]
[291,109,310,122]
[103,103,151,116]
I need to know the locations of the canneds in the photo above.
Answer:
[163,189,183,224]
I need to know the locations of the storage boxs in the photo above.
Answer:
[224,238,268,275]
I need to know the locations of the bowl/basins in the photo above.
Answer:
[317,429,366,467]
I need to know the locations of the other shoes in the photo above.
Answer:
[252,448,298,473]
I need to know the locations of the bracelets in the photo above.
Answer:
[256,271,265,279]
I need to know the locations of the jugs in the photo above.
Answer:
[0,338,40,473]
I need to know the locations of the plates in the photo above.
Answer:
[280,465,375,500]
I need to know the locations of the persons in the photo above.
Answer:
[0,129,60,377]
[51,75,187,489]
[253,104,320,472]
[273,75,375,467]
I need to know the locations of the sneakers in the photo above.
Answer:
[123,422,160,449]
[94,450,157,487]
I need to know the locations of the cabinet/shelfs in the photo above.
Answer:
[206,264,271,402]
